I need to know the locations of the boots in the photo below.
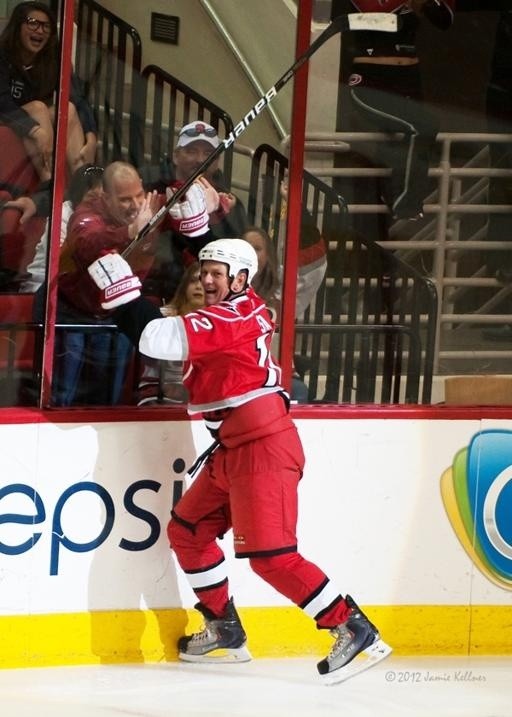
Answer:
[317,594,381,674]
[176,595,247,656]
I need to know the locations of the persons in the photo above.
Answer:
[137,238,391,676]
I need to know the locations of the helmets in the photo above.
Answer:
[199,237,259,286]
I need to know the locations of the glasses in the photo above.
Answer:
[25,15,52,35]
[178,126,217,138]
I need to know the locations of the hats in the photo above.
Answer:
[172,120,219,166]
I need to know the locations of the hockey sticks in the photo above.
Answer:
[120,13,398,260]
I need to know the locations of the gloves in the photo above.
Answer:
[87,247,143,311]
[167,182,211,238]
